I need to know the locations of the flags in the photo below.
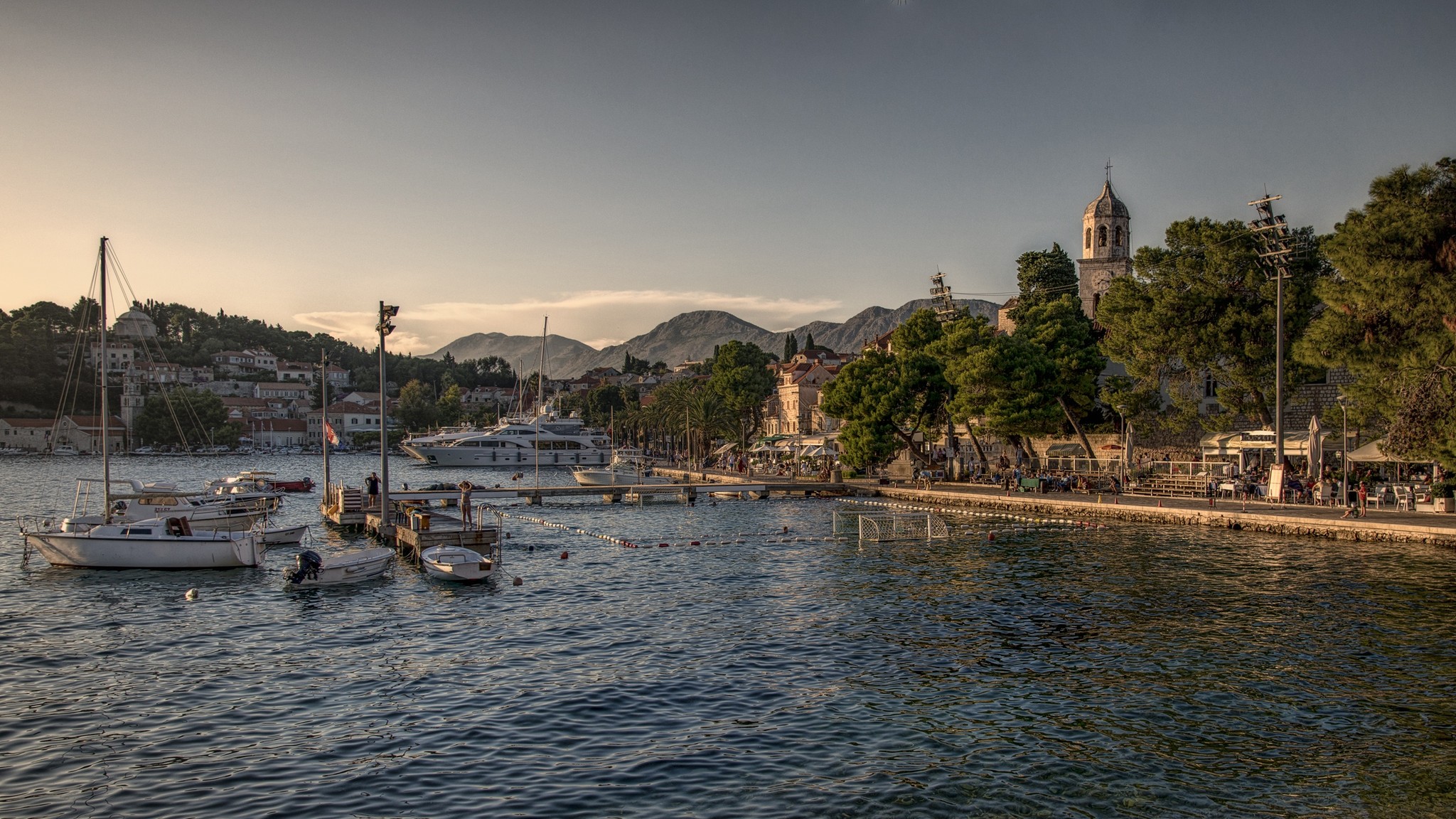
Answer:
[326,420,341,446]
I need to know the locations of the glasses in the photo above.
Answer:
[463,483,465,485]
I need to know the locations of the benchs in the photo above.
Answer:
[1122,474,1228,498]
[407,513,431,529]
[803,468,812,476]
[969,467,1105,495]
[752,464,778,474]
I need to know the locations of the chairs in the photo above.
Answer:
[1210,475,1432,511]
[392,501,413,524]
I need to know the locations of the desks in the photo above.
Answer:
[1348,490,1361,509]
[1256,486,1268,501]
[1019,479,1047,493]
[1219,484,1242,500]
[1405,492,1425,511]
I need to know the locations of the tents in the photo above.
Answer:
[1347,431,1446,475]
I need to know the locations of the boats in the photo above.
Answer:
[282,545,397,586]
[125,468,317,544]
[562,404,676,487]
[236,314,620,467]
[420,543,497,582]
[53,444,79,456]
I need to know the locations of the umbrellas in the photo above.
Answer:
[1101,444,1125,451]
[714,442,839,469]
[1124,421,1136,474]
[1307,414,1322,480]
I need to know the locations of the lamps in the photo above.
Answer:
[402,483,408,490]
[1335,450,1342,458]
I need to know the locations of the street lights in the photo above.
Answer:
[129,427,133,452]
[288,427,291,448]
[1116,404,1128,492]
[373,300,400,539]
[653,428,658,449]
[740,418,747,455]
[211,427,214,448]
[320,348,335,504]
[795,416,805,476]
[1336,394,1352,507]
[662,427,667,450]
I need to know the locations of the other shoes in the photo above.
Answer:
[372,505,377,508]
[368,506,373,508]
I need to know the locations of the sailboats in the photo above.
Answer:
[16,238,283,570]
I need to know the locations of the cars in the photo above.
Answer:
[184,444,231,453]
[135,446,154,452]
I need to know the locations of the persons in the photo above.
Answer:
[458,481,474,530]
[647,447,835,481]
[911,443,1445,518]
[365,472,382,508]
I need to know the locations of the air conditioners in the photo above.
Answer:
[800,413,812,420]
[773,389,778,394]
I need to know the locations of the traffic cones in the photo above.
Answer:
[1097,495,1102,504]
[1006,490,1010,497]
[1157,499,1163,507]
[893,483,897,488]
[916,484,920,490]
[1114,497,1119,504]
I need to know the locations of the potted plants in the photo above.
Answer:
[1432,482,1454,512]
